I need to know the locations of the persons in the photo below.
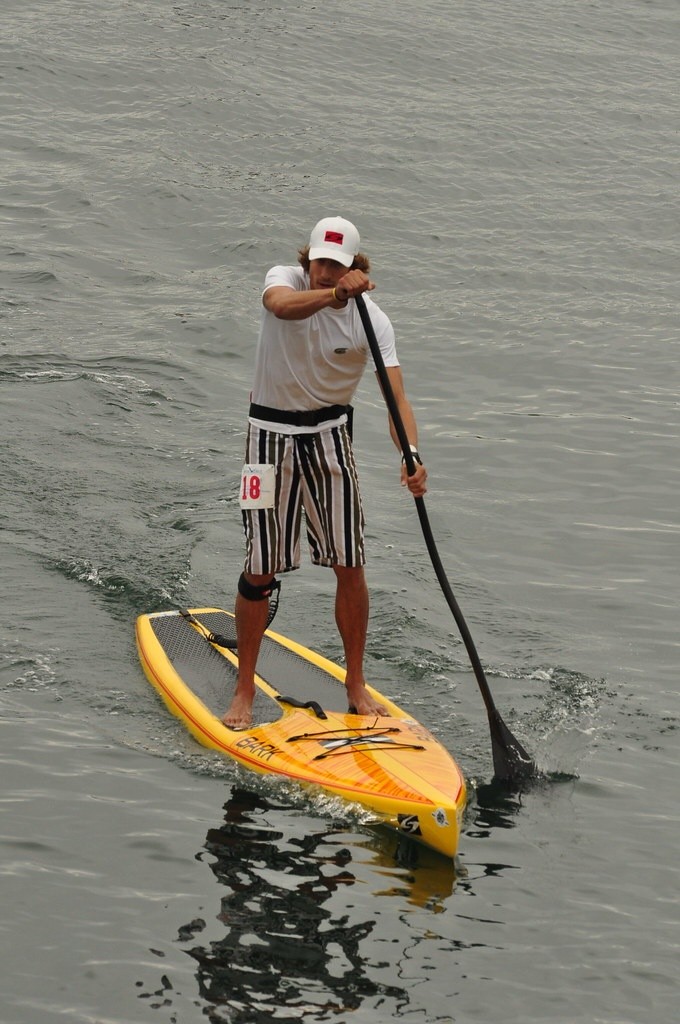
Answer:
[223,217,429,730]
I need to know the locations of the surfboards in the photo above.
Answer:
[133,607,467,859]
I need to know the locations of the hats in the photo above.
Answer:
[304,212,362,271]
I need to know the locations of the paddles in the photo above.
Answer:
[355,294,538,783]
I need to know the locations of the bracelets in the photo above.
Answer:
[401,452,423,466]
[333,287,348,304]
